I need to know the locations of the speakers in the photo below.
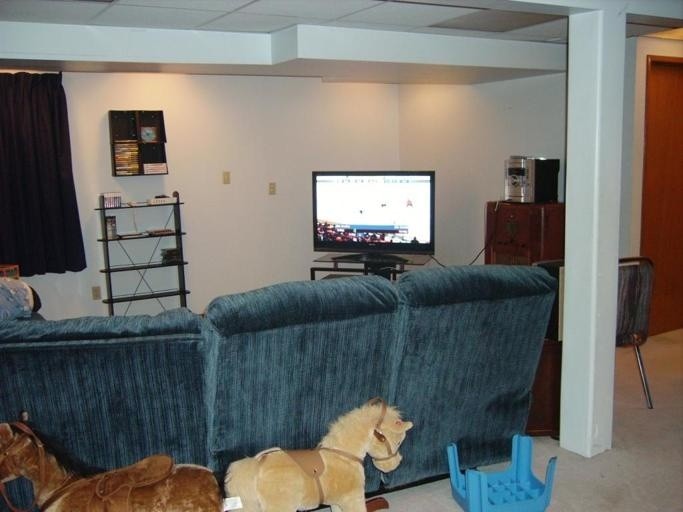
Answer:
[504,158,560,203]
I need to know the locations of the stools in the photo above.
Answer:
[446,433,557,512]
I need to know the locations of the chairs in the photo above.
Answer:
[533,260,564,279]
[615,257,655,408]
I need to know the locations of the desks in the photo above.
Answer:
[525,340,561,438]
[310,253,434,281]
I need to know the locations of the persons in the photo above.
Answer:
[316,218,420,245]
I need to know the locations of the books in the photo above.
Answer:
[113,138,140,176]
[143,162,167,175]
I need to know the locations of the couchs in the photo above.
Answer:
[0,266,558,512]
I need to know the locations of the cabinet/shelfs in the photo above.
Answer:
[484,200,564,265]
[94,192,189,318]
[108,108,169,176]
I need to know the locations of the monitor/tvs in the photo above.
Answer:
[311,170,436,263]
